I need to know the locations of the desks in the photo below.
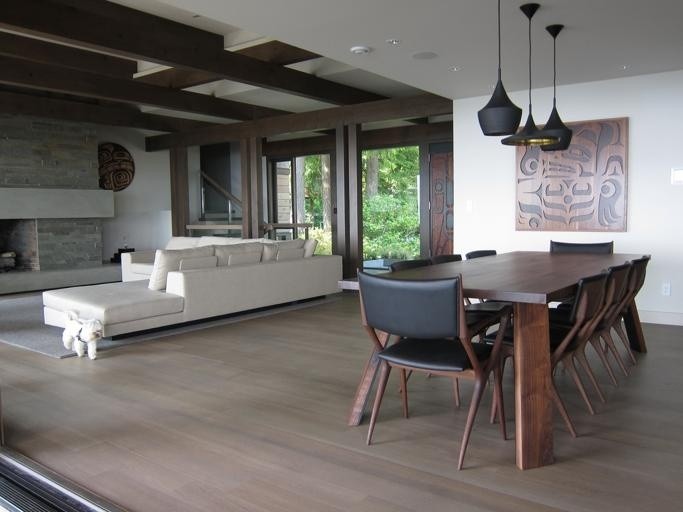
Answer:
[337,251,651,470]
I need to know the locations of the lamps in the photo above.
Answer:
[476,0,573,152]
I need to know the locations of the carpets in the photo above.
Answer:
[0,294,342,361]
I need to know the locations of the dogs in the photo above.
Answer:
[62,311,103,360]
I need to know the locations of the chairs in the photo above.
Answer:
[353,267,509,466]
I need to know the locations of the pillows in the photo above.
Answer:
[148,245,215,291]
[262,238,304,262]
[215,241,261,266]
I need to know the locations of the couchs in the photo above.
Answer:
[41,236,344,337]
